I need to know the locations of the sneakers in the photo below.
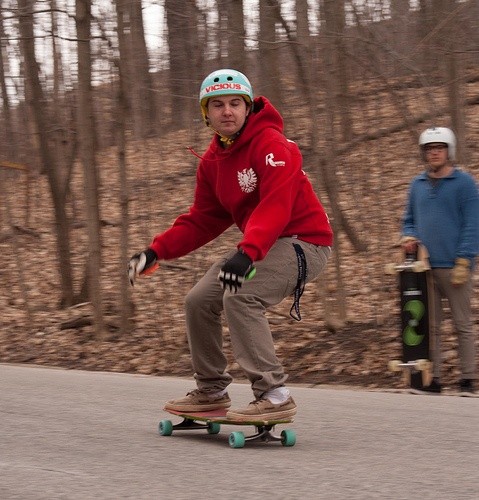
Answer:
[165,388,231,411]
[227,396,296,422]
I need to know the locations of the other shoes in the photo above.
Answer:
[457,380,474,397]
[415,379,441,392]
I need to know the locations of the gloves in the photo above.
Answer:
[218,247,252,295]
[127,249,157,288]
[451,257,470,288]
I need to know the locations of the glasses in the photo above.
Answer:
[422,144,448,153]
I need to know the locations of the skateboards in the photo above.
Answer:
[158,408,296,448]
[383,244,435,388]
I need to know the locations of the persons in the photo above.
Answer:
[400,125,478,398]
[128,69,333,421]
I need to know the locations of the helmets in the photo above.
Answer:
[200,68,254,127]
[419,126,457,161]
